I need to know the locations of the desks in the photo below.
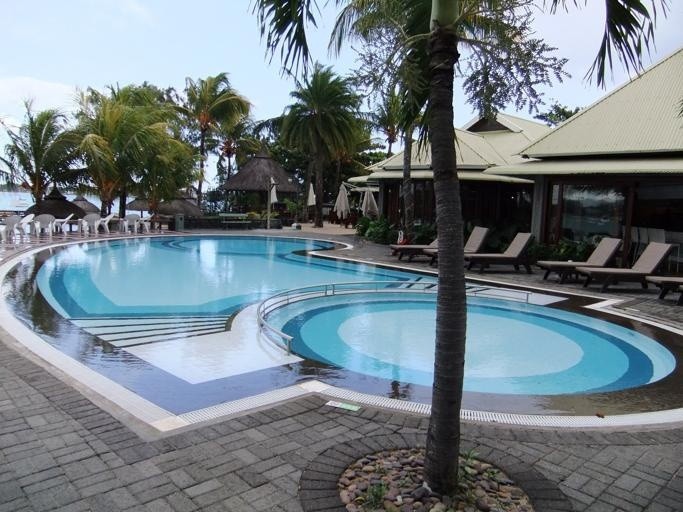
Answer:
[219,213,248,230]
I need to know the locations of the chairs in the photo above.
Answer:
[537,236,622,284]
[464,232,535,273]
[621,225,683,273]
[676,285,683,306]
[423,226,489,267]
[644,275,683,300]
[576,242,673,293]
[0,213,155,249]
[389,238,438,263]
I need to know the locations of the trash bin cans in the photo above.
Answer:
[150,214,161,233]
[175,213,183,232]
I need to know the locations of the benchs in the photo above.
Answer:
[221,220,252,224]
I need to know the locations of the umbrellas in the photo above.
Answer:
[306,181,316,206]
[361,184,381,220]
[333,182,350,222]
[268,178,279,210]
